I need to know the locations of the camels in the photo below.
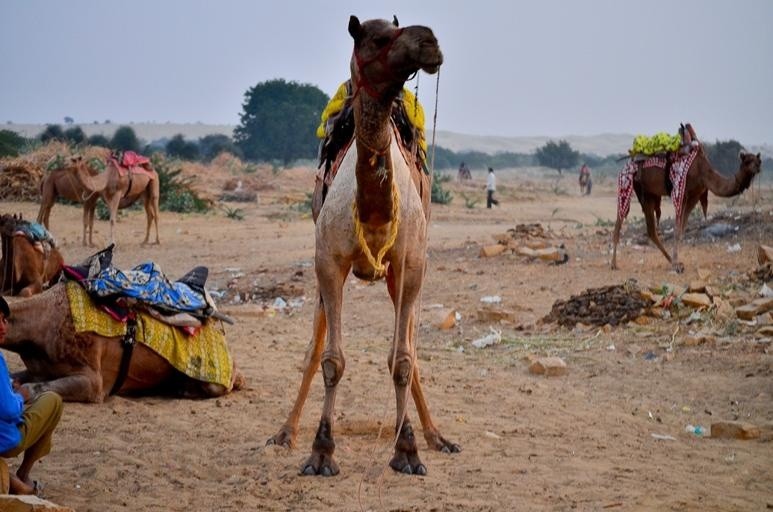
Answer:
[0,208,246,405]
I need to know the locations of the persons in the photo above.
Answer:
[580,164,590,180]
[0,296,65,497]
[482,167,499,209]
